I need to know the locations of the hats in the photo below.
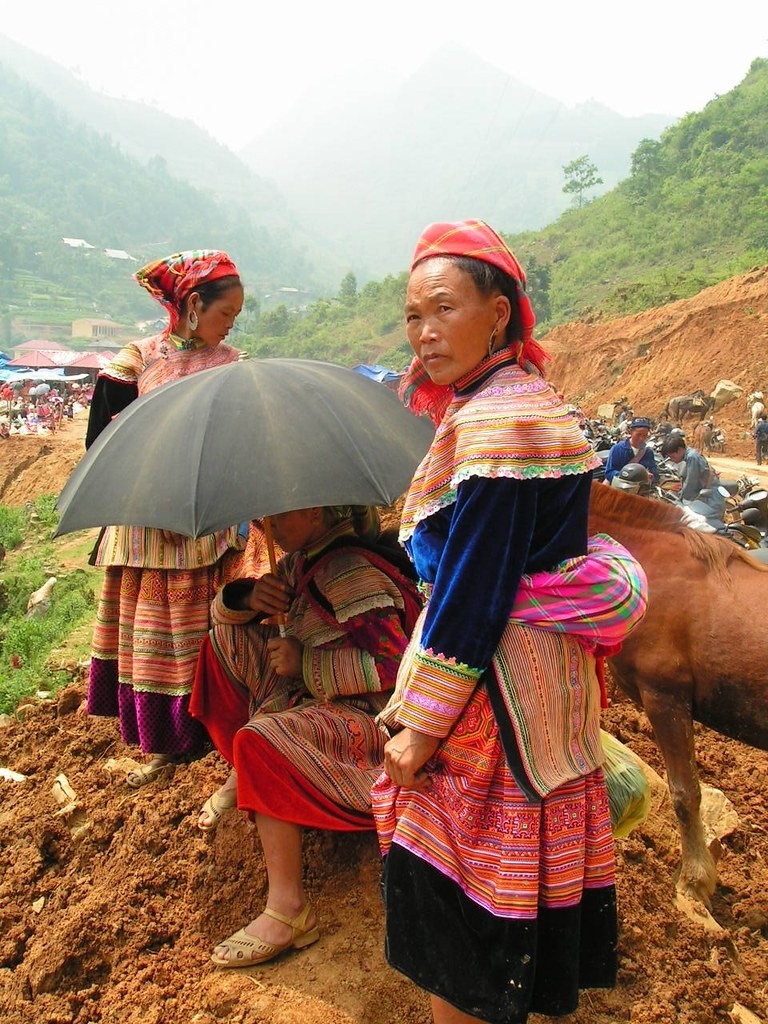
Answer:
[399,217,548,430]
[133,247,239,341]
[631,417,650,429]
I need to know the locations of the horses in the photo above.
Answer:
[691,424,712,457]
[570,480,768,914]
[664,396,716,424]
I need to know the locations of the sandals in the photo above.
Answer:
[210,900,321,966]
[197,770,237,830]
[127,756,173,787]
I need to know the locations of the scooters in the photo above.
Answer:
[702,416,727,454]
[717,479,768,564]
[654,476,761,550]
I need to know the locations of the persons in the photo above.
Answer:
[184,503,429,969]
[617,405,672,435]
[379,221,623,1023]
[1,384,95,437]
[86,248,266,791]
[753,414,768,466]
[601,415,660,495]
[662,436,726,521]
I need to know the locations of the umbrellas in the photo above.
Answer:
[46,351,442,639]
[1,368,88,384]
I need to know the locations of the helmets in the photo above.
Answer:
[611,461,651,496]
